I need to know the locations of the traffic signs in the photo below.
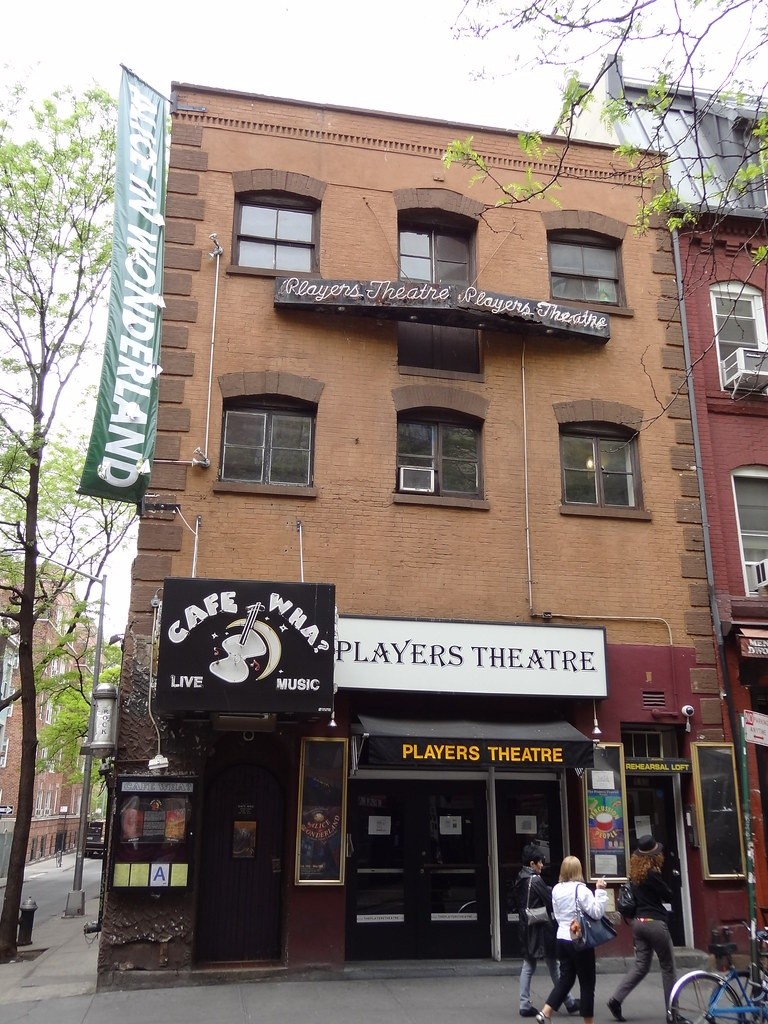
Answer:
[0,804,15,816]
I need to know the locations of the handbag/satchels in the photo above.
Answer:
[618,879,636,918]
[570,884,618,952]
[526,906,549,924]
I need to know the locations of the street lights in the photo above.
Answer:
[0,550,107,891]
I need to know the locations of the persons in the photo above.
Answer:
[519,844,580,1017]
[536,856,606,1024]
[606,835,693,1024]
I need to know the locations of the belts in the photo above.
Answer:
[637,917,653,922]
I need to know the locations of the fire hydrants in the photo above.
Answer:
[17,895,39,947]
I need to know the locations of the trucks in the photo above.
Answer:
[84,818,106,860]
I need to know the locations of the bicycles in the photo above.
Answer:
[666,919,768,1024]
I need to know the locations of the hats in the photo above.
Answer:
[634,835,663,855]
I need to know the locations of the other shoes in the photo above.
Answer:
[666,1015,693,1024]
[566,999,581,1013]
[607,998,626,1021]
[519,1006,539,1016]
[536,1011,551,1024]
